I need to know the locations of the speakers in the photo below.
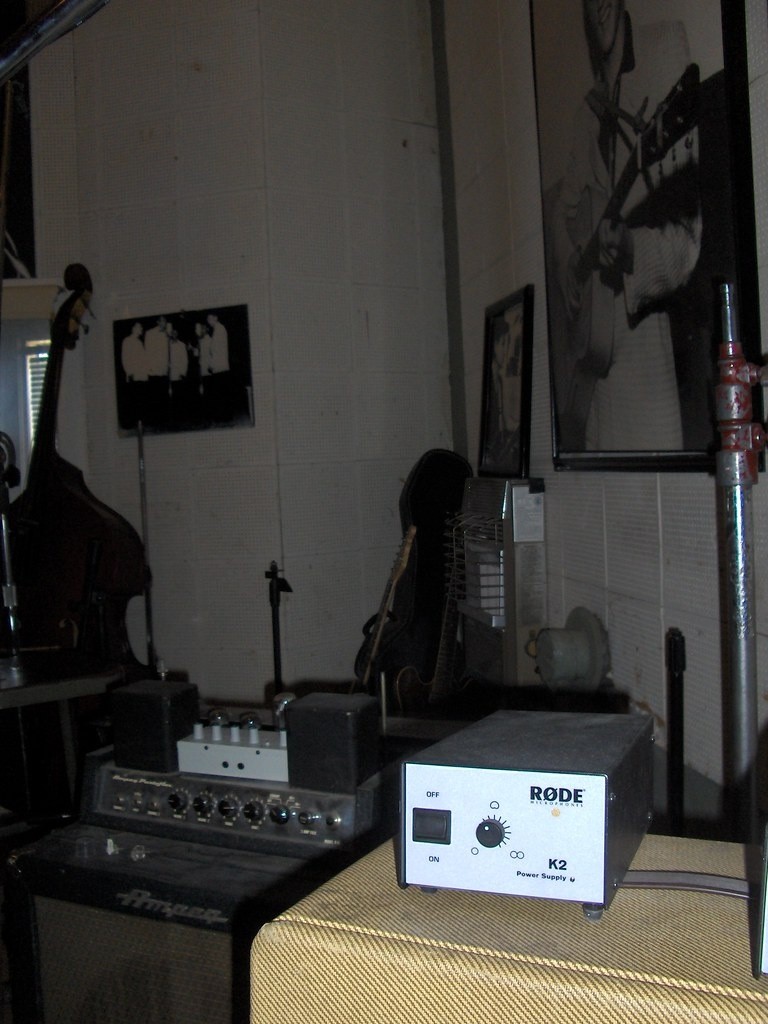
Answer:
[0,821,343,1024]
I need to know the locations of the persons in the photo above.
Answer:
[550,0,705,450]
[121,313,234,425]
[486,319,512,445]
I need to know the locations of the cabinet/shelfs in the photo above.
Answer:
[442,476,549,686]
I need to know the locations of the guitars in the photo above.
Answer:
[551,65,705,438]
[358,523,417,690]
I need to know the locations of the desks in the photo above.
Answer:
[1,648,126,817]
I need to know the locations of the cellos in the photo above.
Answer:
[2,261,152,828]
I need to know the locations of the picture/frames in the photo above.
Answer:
[476,283,535,478]
[528,0,767,472]
